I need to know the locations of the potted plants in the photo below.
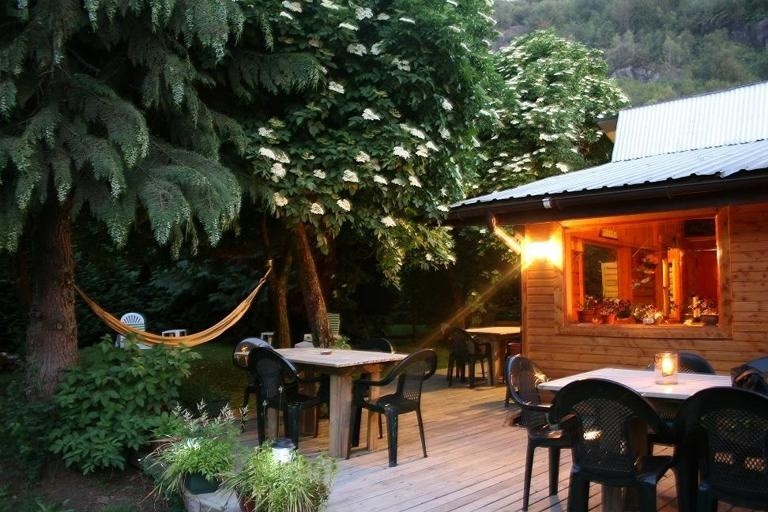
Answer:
[578,295,719,325]
[207,441,337,512]
[141,398,250,503]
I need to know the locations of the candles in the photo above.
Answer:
[660,353,674,377]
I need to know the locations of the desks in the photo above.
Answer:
[464,326,521,384]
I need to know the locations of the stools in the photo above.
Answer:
[261,332,275,344]
[162,329,187,337]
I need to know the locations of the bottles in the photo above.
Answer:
[271,438,296,463]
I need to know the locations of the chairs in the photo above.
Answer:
[241,337,438,467]
[647,353,716,446]
[444,327,494,388]
[549,378,684,512]
[507,354,569,512]
[115,313,152,349]
[731,369,767,396]
[675,386,767,512]
